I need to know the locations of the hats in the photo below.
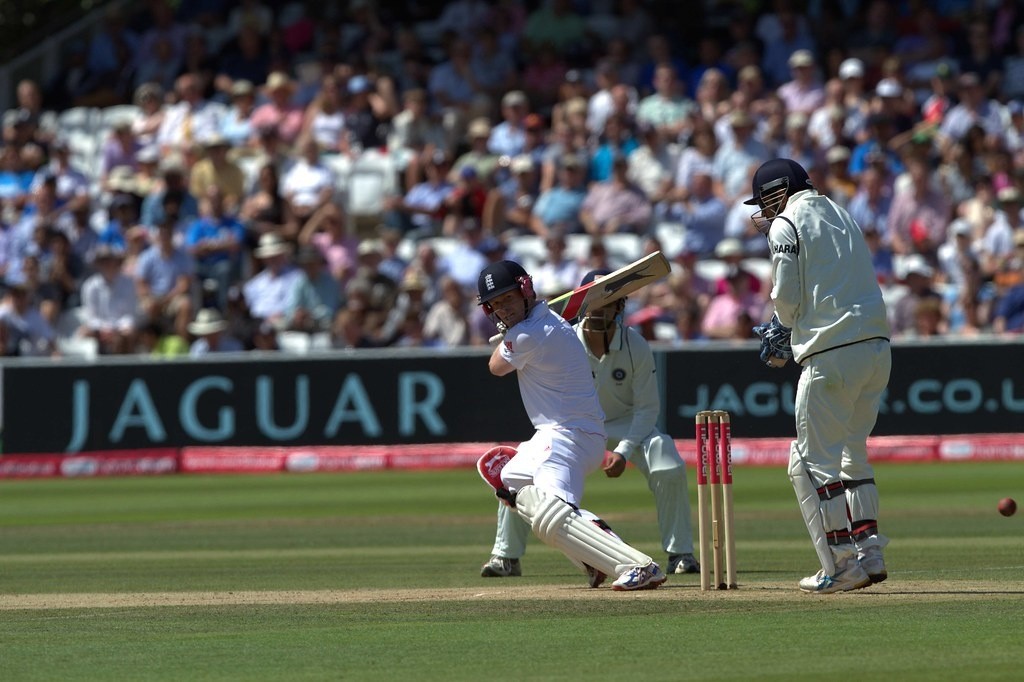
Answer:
[0,0,1024,341]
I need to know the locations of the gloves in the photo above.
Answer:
[752,310,794,368]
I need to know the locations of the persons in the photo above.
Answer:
[0,50,1024,357]
[478,260,667,590]
[481,268,700,575]
[743,158,890,593]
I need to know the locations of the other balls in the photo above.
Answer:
[998,496,1017,517]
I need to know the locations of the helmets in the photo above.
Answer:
[578,270,628,332]
[476,260,535,332]
[743,158,814,237]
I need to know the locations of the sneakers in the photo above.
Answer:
[798,558,871,594]
[859,544,887,583]
[666,553,700,574]
[481,555,522,576]
[589,568,608,588]
[611,562,668,591]
